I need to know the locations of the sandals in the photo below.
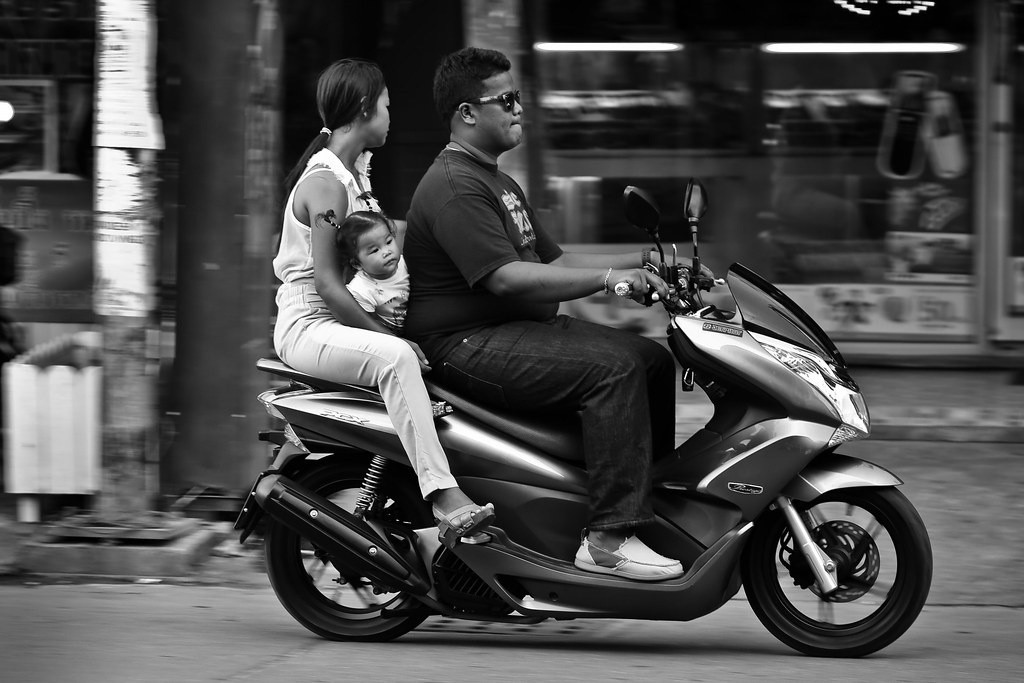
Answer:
[432,495,496,549]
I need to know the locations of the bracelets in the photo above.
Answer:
[604,267,613,294]
[641,246,658,272]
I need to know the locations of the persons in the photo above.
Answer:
[314,192,454,420]
[272,59,494,527]
[401,47,715,581]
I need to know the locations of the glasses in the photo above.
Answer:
[467,89,522,113]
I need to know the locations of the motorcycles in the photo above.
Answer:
[231,179,936,664]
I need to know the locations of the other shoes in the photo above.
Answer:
[432,400,454,420]
[575,534,684,580]
